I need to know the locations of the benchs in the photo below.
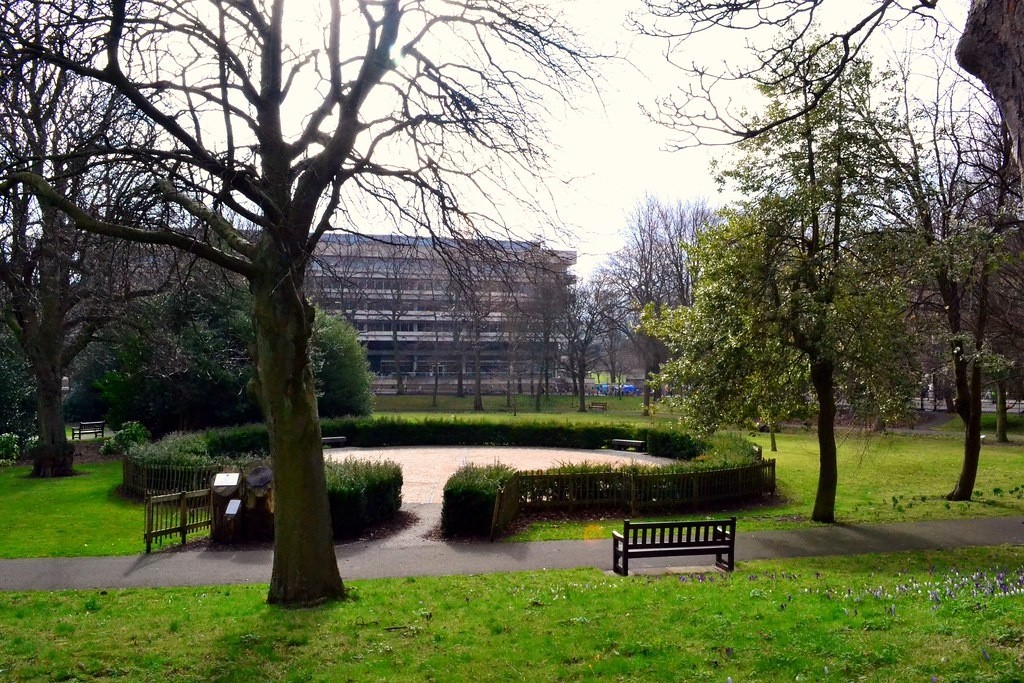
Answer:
[612,516,737,576]
[321,436,346,448]
[589,401,607,411]
[71,420,105,440]
[611,438,646,453]
